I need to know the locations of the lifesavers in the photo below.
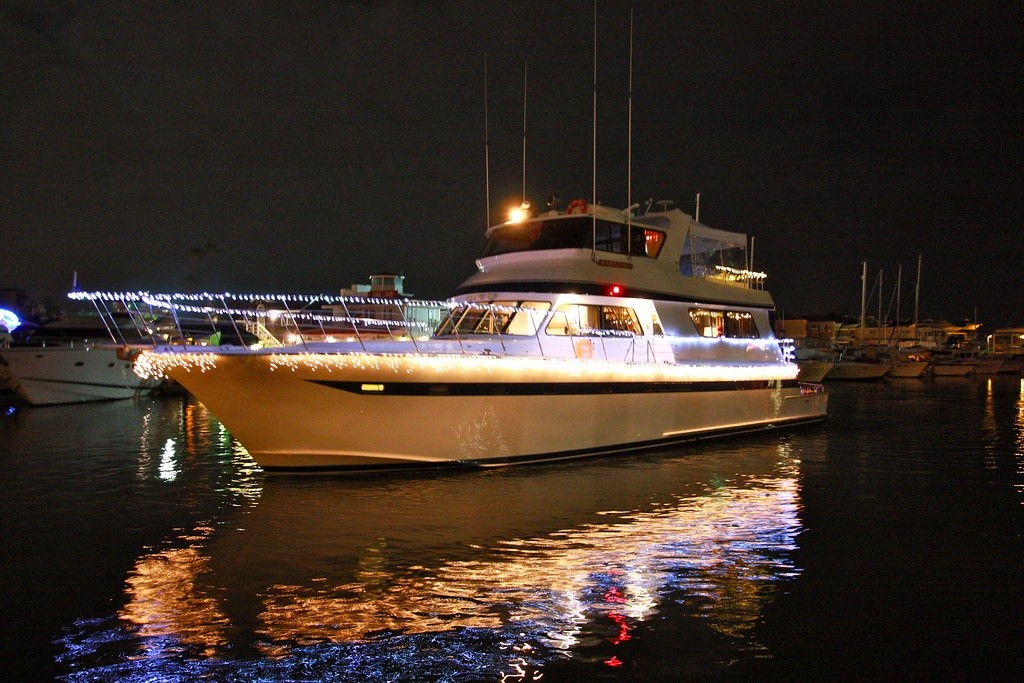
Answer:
[566,199,587,215]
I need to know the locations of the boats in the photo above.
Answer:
[789,349,1024,382]
[0,313,258,405]
[69,192,828,473]
